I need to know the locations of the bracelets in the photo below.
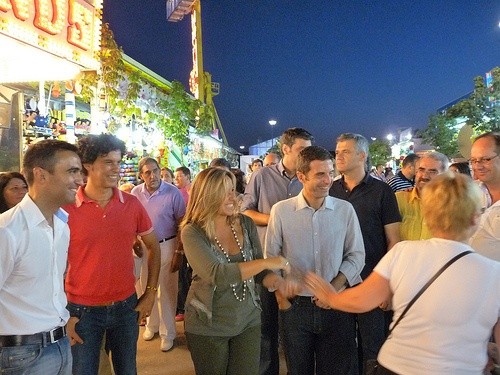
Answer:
[174,249,184,256]
[146,286,157,292]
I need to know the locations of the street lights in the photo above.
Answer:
[239,145,245,154]
[268,120,278,147]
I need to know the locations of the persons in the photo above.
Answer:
[0,139,84,375]
[265,146,366,375]
[0,172,29,215]
[369,150,493,245]
[123,158,194,351]
[463,131,500,266]
[329,133,399,375]
[181,168,291,375]
[300,172,500,375]
[211,144,283,195]
[64,135,162,375]
[240,127,315,375]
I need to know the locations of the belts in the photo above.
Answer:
[159,235,177,243]
[0,325,67,348]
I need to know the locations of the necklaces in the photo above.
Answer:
[200,217,249,302]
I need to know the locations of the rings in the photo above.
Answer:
[285,262,289,265]
[137,246,140,249]
[145,313,149,315]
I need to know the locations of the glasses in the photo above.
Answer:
[416,168,441,177]
[469,152,500,167]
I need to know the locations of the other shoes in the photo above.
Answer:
[161,338,173,351]
[143,329,154,341]
[175,314,184,321]
